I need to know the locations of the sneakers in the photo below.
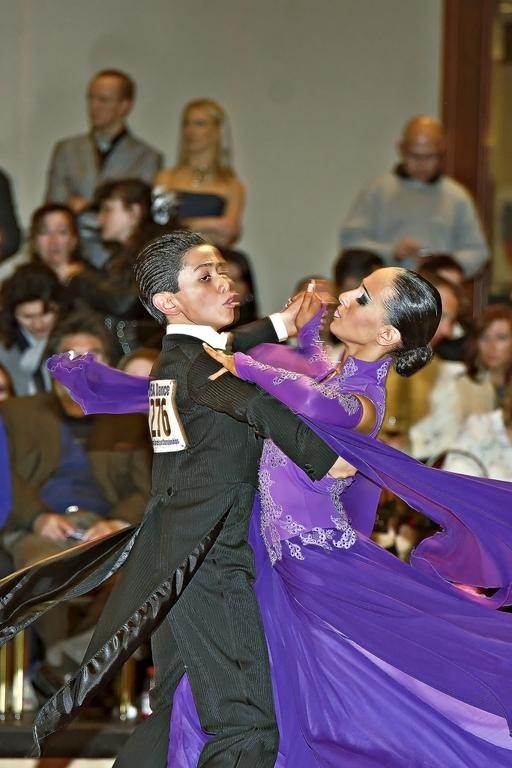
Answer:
[30,661,79,699]
